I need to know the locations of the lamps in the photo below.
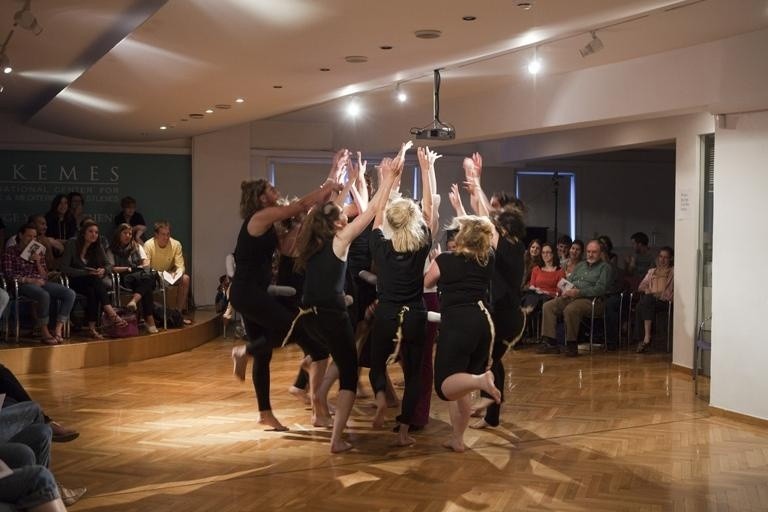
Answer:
[0,48,13,76]
[392,82,410,105]
[525,45,545,79]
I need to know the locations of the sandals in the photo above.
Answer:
[42,300,192,344]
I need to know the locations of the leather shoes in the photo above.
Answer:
[61,487,87,507]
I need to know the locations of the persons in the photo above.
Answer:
[214,140,673,454]
[0,193,191,344]
[0,363,87,511]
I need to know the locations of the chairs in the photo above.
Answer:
[694,313,714,397]
[0,265,189,342]
[519,282,673,352]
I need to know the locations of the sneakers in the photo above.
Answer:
[521,336,652,356]
[50,422,80,442]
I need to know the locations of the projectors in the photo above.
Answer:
[416,129,456,140]
